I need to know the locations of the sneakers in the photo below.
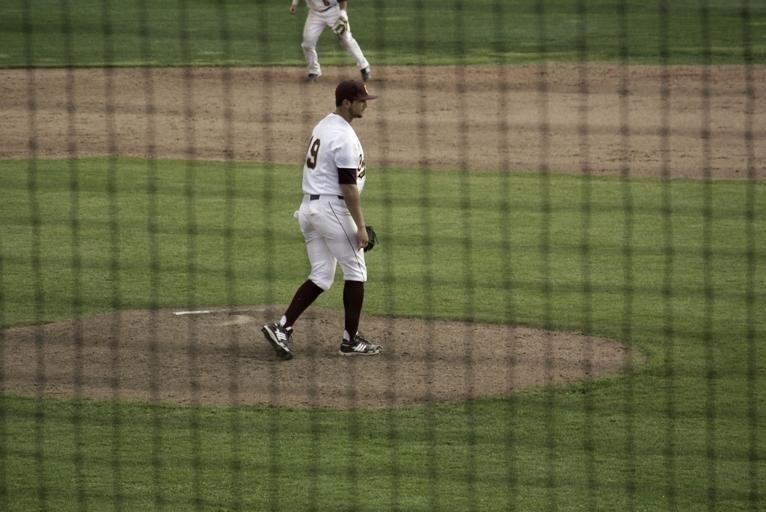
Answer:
[361,66,370,82]
[305,72,322,81]
[262,314,294,360]
[339,335,383,356]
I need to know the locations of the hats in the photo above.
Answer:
[336,80,378,101]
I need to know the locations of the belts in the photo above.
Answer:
[310,193,344,199]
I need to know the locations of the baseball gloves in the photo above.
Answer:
[332,16,347,39]
[363,225,377,252]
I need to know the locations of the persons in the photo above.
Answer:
[289,0,371,81]
[261,80,383,359]
